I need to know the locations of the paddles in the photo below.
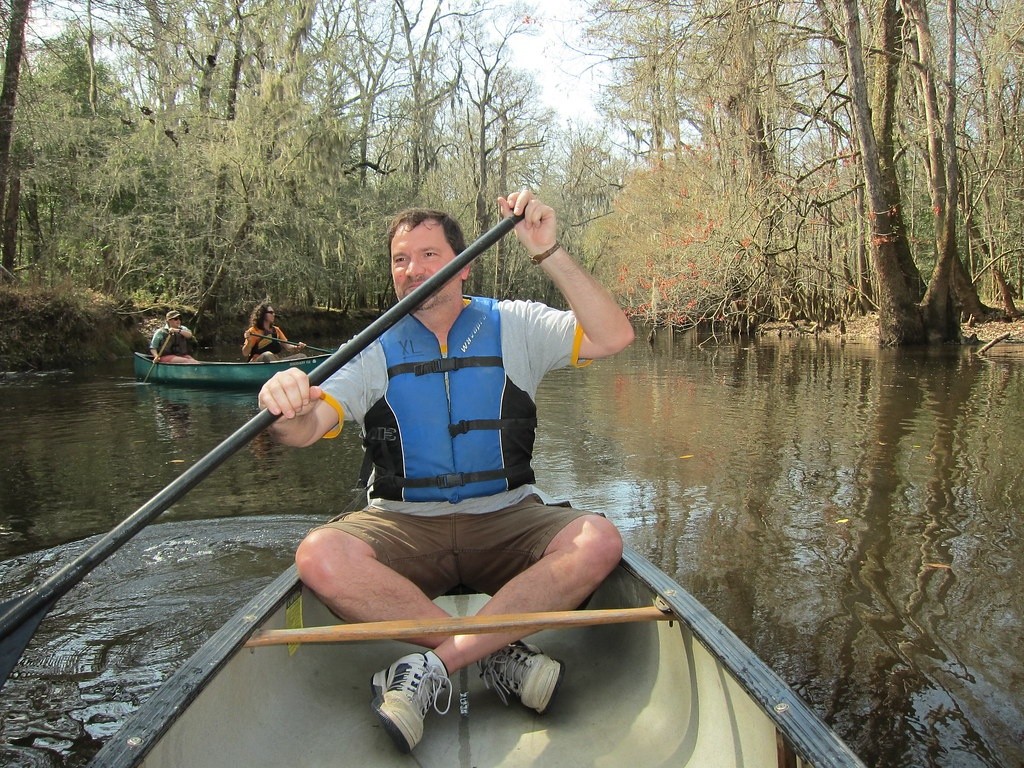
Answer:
[144,332,172,383]
[249,330,337,353]
[0,205,529,684]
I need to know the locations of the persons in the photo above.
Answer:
[150,310,199,364]
[256,189,636,756]
[241,302,308,362]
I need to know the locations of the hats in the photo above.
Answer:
[166,310,180,320]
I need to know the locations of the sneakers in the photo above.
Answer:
[476,638,566,716]
[370,652,452,753]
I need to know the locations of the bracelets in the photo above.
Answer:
[526,239,563,267]
[177,329,182,335]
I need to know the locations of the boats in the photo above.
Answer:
[133,352,333,389]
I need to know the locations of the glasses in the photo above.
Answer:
[266,311,276,314]
[171,317,183,320]
